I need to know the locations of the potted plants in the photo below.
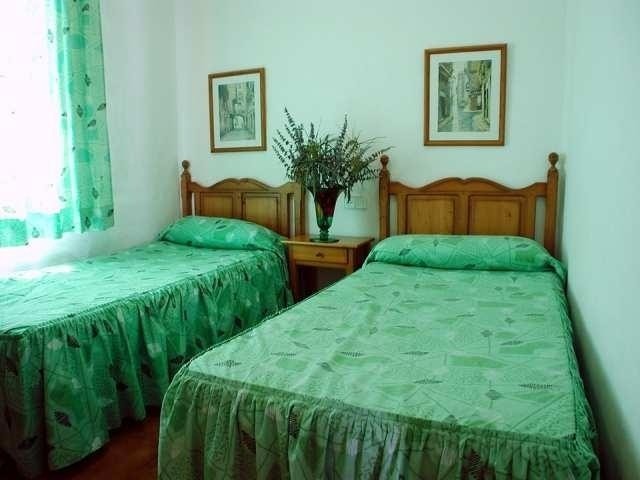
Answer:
[272,105,394,243]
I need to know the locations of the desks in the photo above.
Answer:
[280,231,374,301]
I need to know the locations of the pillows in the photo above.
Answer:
[154,216,287,259]
[361,233,566,275]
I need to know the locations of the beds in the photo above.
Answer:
[159,153,600,480]
[0,160,306,480]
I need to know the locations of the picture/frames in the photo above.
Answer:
[424,43,506,146]
[208,67,266,153]
